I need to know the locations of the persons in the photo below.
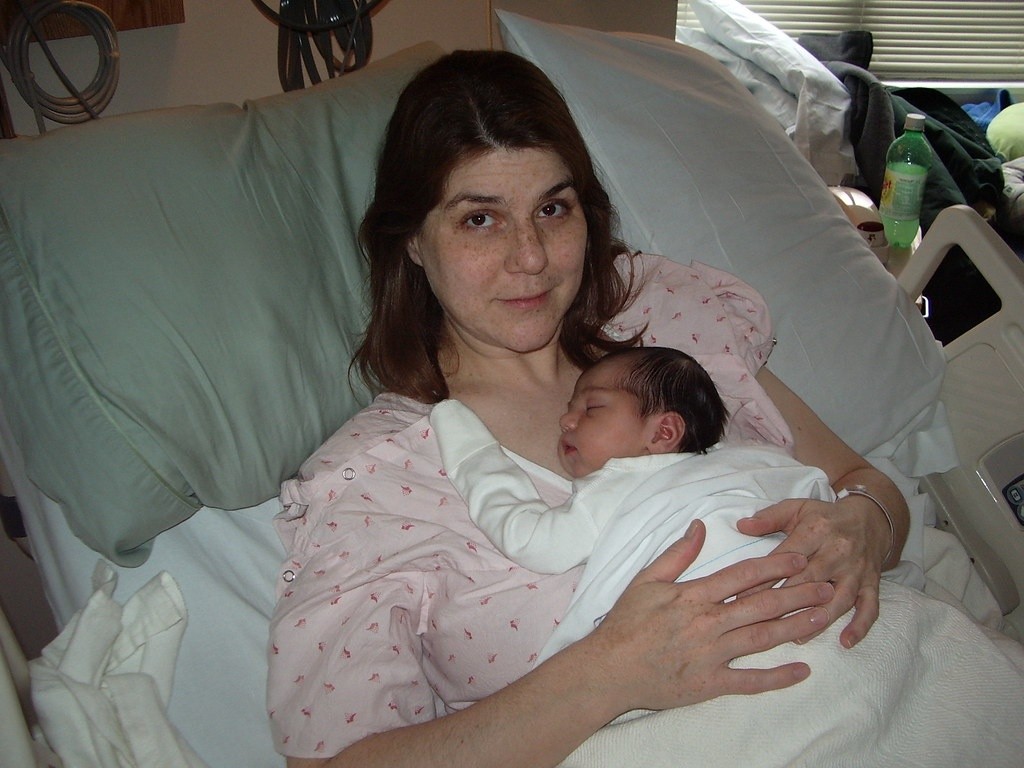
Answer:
[429,348,731,576]
[264,51,912,768]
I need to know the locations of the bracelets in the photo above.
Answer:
[836,490,896,567]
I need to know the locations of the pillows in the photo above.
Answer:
[0,39,451,568]
[495,7,960,477]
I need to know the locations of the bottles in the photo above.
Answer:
[878,114,933,248]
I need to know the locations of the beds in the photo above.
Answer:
[0,204,1024,768]
